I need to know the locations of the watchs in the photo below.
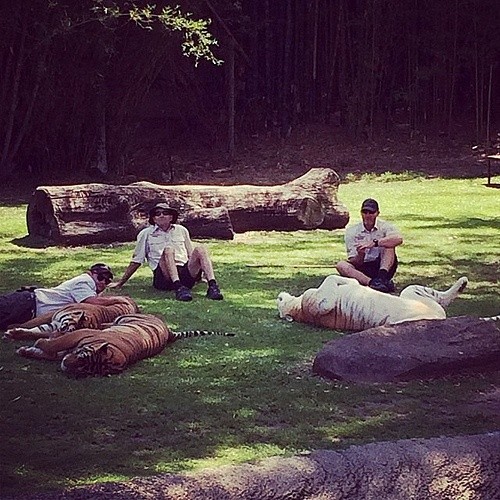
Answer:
[373,238,379,247]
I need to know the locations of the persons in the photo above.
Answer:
[107,203,225,302]
[336,198,403,293]
[1,263,131,331]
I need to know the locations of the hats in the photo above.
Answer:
[91,263,114,280]
[148,203,178,226]
[360,198,378,212]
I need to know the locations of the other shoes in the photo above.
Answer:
[384,280,395,292]
[206,286,223,299]
[175,286,192,302]
[369,279,388,293]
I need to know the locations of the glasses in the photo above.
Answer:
[152,210,173,216]
[91,271,111,285]
[361,210,377,214]
